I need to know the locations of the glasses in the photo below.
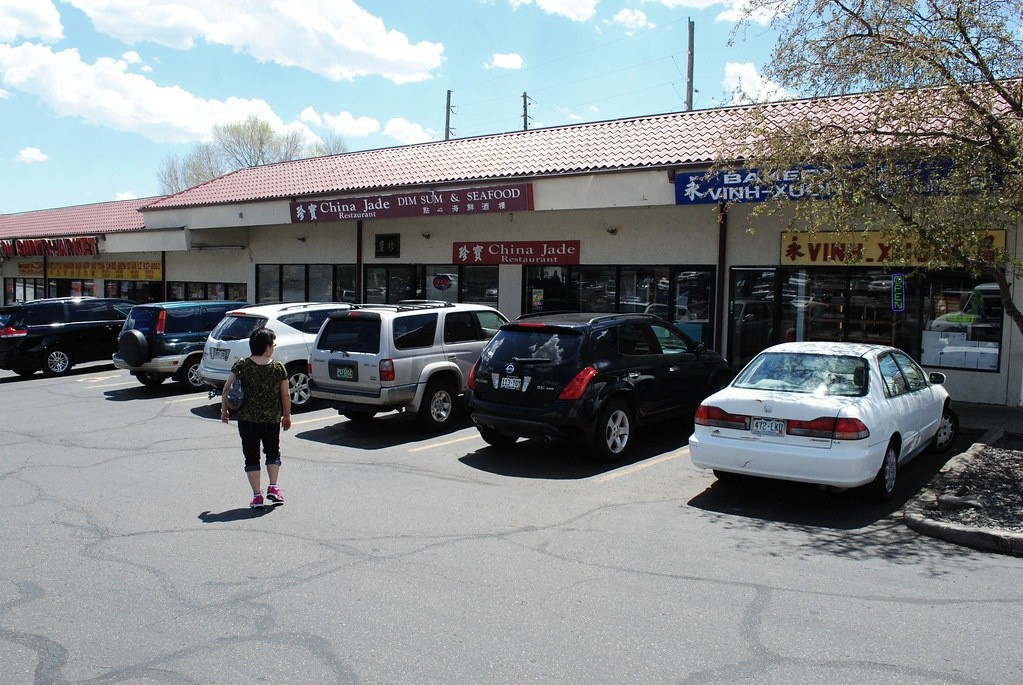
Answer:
[272,342,276,346]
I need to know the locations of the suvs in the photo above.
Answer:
[112,299,255,394]
[306,299,511,434]
[0,296,146,378]
[197,302,365,415]
[465,310,737,468]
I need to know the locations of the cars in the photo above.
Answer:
[689,341,962,504]
[334,276,1008,349]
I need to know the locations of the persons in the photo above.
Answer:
[220,327,291,506]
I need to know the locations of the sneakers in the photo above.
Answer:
[266,486,283,502]
[249,495,264,507]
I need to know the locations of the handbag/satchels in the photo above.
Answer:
[226,358,245,410]
